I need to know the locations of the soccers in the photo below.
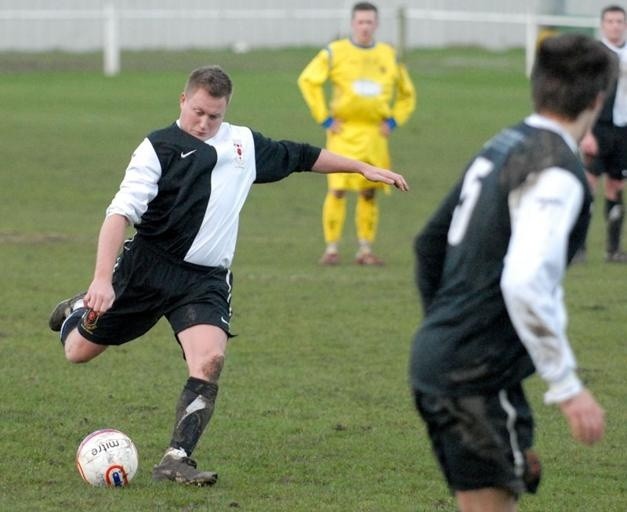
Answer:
[76,429,138,487]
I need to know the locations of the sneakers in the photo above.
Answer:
[150,452,220,490]
[568,246,627,269]
[315,246,385,270]
[48,290,87,334]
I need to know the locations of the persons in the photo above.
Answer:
[562,4,625,262]
[48,64,411,483]
[410,36,618,509]
[295,2,418,265]
